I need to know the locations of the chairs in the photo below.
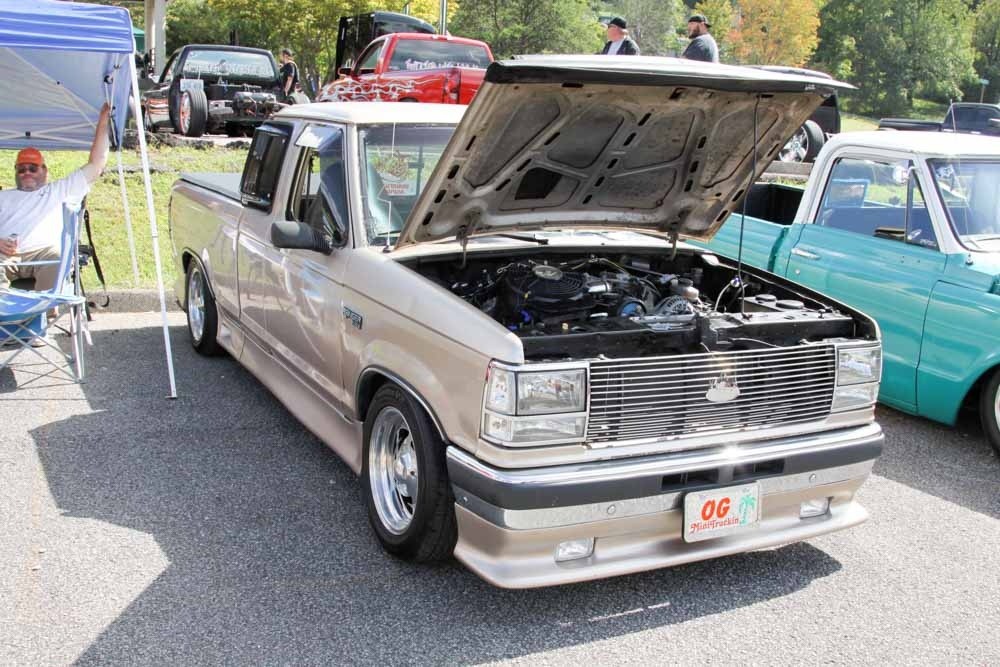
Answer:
[0,204,111,383]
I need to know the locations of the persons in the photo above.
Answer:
[0,101,111,347]
[280,48,299,96]
[595,16,640,55]
[681,15,720,64]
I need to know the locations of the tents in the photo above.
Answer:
[0,0,179,399]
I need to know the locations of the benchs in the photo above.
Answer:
[819,204,991,238]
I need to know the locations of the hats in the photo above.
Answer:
[16,148,43,166]
[610,17,626,28]
[689,15,712,28]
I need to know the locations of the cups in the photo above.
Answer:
[8,233,19,249]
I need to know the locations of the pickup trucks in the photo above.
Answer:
[879,103,1000,135]
[681,127,1000,456]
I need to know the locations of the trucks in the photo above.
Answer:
[175,0,451,135]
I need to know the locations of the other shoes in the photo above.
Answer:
[1,339,17,345]
[27,336,48,347]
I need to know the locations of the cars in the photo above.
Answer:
[136,44,277,136]
[313,32,501,107]
[163,50,889,592]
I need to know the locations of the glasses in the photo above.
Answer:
[608,24,616,28]
[18,165,38,174]
